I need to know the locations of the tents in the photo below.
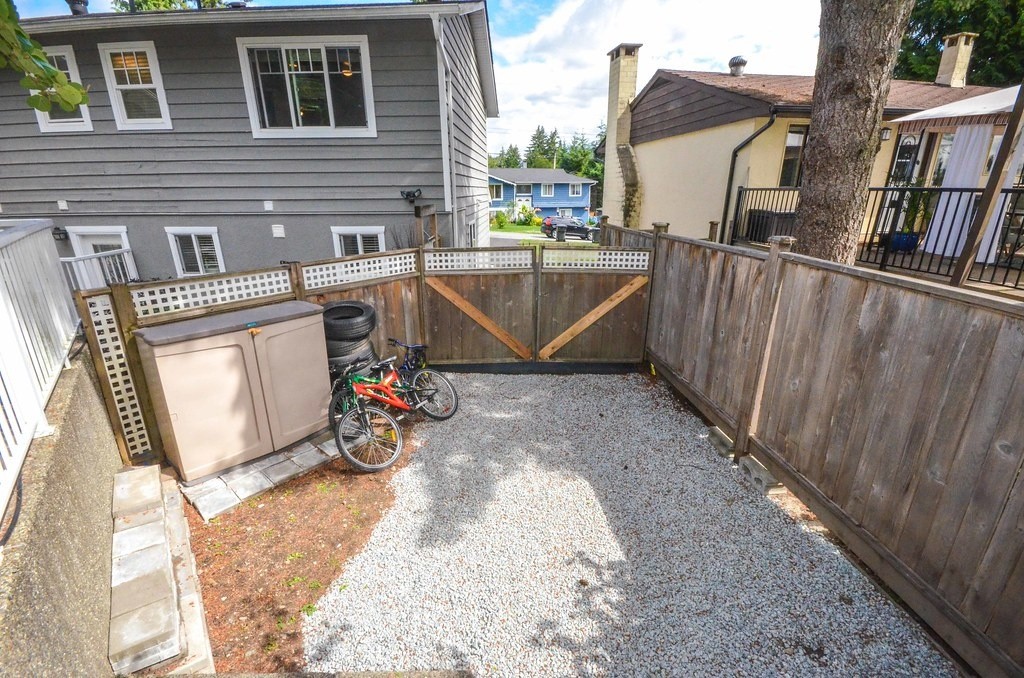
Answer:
[886,83,1024,263]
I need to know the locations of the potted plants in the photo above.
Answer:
[890,176,939,256]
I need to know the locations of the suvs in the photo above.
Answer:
[540,215,601,241]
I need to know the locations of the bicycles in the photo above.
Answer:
[387,337,430,411]
[328,357,428,442]
[334,354,458,473]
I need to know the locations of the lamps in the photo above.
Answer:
[52,226,68,241]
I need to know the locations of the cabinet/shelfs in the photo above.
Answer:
[128,300,334,487]
